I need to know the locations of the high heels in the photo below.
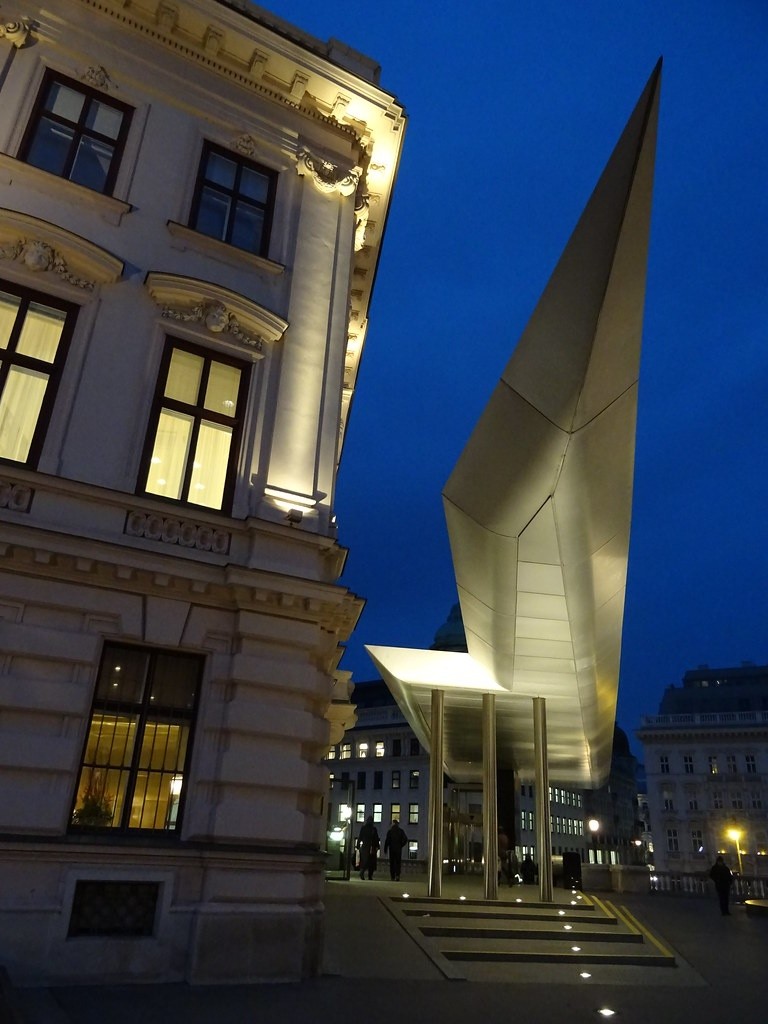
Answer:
[391,876,395,880]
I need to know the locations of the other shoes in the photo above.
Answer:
[721,912,733,916]
[359,872,364,880]
[396,876,400,881]
[368,875,373,880]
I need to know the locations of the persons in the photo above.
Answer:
[384,820,407,881]
[506,850,518,873]
[357,816,380,880]
[712,856,732,915]
[465,824,482,862]
[521,854,538,885]
[498,826,509,863]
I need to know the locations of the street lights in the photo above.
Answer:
[731,830,743,875]
[589,819,599,864]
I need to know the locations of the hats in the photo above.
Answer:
[525,853,531,857]
[366,816,373,821]
[715,856,723,863]
[391,820,400,826]
